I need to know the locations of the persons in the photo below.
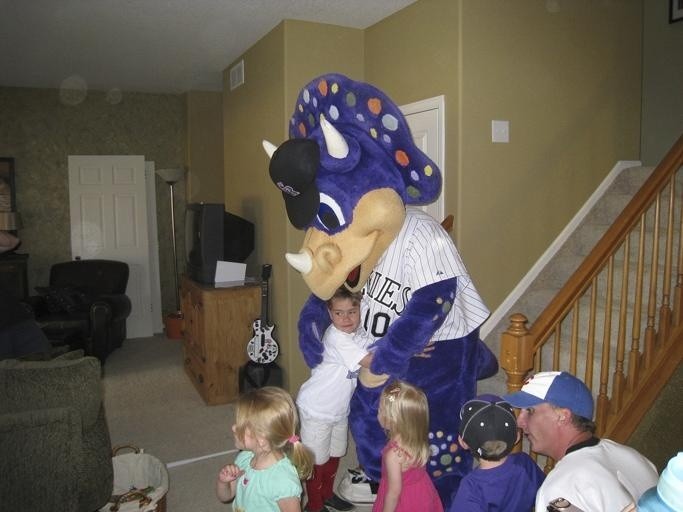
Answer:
[216,385,315,512]
[295,286,435,512]
[637,451,683,511]
[371,383,444,512]
[502,371,659,511]
[448,395,546,512]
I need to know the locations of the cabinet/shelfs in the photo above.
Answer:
[179,272,268,407]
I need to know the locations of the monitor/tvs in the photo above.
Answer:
[185,203,256,286]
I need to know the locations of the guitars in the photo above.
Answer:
[247,265,279,364]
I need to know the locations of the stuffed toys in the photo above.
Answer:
[262,72,499,512]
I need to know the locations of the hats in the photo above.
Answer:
[503,371,594,422]
[456,393,519,460]
[268,139,319,228]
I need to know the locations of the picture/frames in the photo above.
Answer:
[668,0,683,23]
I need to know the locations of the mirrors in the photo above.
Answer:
[0,157,16,253]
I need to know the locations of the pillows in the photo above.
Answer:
[49,282,97,319]
[33,286,66,314]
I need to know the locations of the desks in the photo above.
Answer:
[0,253,29,301]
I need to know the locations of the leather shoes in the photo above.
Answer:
[326,496,354,510]
[305,506,331,512]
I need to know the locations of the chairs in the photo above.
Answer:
[0,299,113,512]
[22,260,131,365]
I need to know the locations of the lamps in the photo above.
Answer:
[155,168,187,340]
[2,212,22,231]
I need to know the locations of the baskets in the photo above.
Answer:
[100,441,169,511]
